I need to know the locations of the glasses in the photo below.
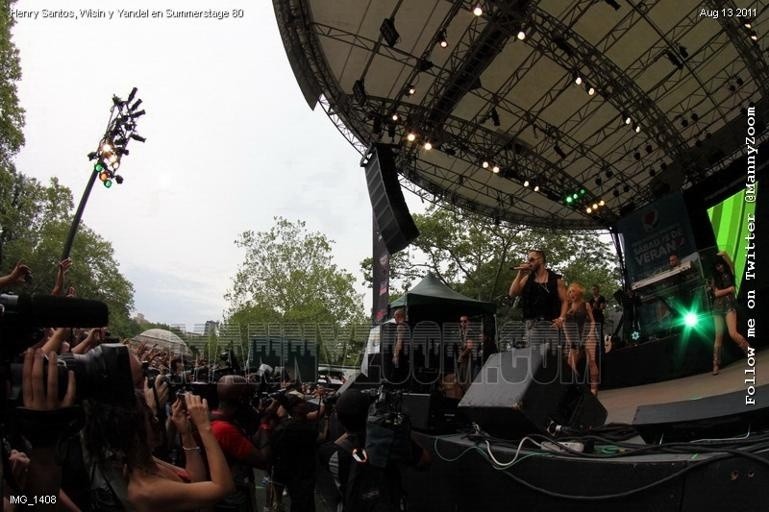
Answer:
[529,256,542,262]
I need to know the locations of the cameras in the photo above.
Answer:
[318,389,336,418]
[141,360,165,400]
[179,380,217,414]
[255,386,288,411]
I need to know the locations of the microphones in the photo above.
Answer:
[509,265,532,273]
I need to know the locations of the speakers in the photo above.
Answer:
[360,142,420,257]
[336,371,369,396]
[399,392,448,433]
[457,346,608,446]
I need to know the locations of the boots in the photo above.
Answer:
[712,346,721,376]
[739,338,749,357]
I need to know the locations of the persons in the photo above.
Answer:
[711,250,755,376]
[669,253,692,318]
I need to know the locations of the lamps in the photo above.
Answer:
[621,112,640,134]
[406,84,416,95]
[351,80,368,108]
[490,107,500,126]
[572,69,595,96]
[553,144,566,160]
[379,19,400,49]
[437,32,448,49]
[472,3,483,17]
